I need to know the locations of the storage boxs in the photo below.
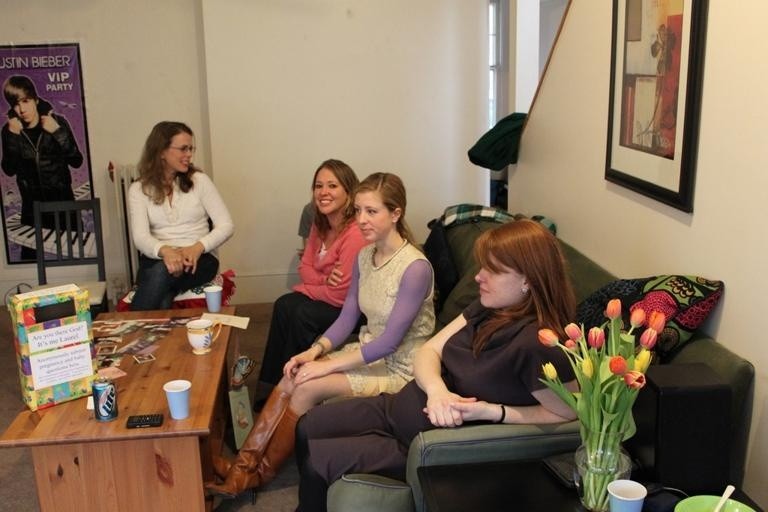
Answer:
[8,283,93,410]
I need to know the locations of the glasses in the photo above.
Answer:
[169,144,196,154]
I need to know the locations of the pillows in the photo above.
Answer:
[575,274,724,367]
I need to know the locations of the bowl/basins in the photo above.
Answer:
[673,495,756,512]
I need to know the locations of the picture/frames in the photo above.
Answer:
[604,0,708,214]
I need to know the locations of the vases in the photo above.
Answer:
[570,420,633,512]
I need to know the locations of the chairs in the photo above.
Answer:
[28,197,112,319]
[113,164,235,308]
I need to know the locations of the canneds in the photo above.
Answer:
[91,376,118,423]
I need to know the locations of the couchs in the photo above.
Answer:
[326,210,756,512]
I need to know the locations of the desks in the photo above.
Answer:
[0,305,241,512]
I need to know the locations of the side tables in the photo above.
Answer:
[416,458,763,512]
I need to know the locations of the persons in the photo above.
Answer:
[0,75,83,260]
[253,158,373,414]
[203,171,436,506]
[295,217,584,511]
[127,120,234,311]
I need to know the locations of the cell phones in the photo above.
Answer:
[126,414,164,428]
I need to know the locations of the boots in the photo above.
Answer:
[207,386,292,505]
[211,410,300,489]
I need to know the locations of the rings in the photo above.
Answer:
[303,373,306,377]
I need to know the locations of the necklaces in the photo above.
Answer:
[368,238,409,272]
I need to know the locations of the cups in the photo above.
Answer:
[162,379,192,420]
[203,285,223,313]
[607,479,647,512]
[186,319,222,355]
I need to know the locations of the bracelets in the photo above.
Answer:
[312,341,325,357]
[495,404,507,425]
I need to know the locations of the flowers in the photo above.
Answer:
[535,294,667,512]
